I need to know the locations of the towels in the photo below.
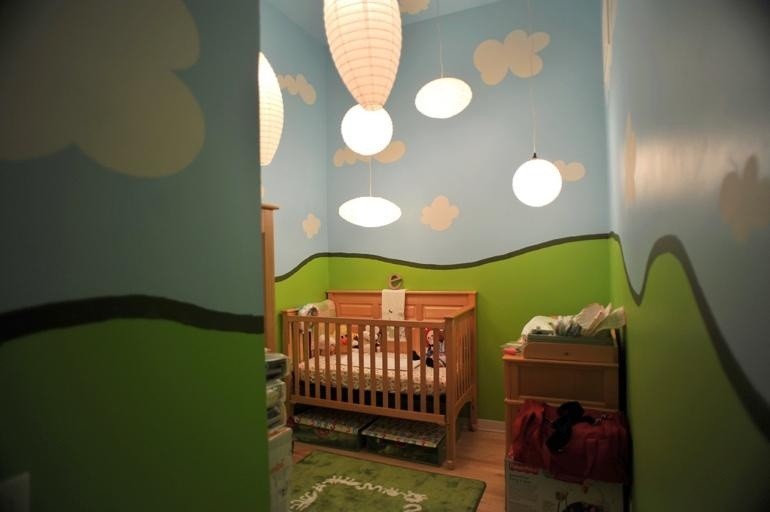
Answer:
[382,288,406,342]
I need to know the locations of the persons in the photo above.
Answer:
[425,327,447,367]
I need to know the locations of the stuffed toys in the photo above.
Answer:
[316,322,383,357]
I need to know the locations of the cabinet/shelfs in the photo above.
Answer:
[261,203,279,353]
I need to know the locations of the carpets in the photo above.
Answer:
[292,449,486,512]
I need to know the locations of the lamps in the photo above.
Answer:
[338,156,401,227]
[340,104,393,156]
[259,51,284,165]
[415,0,472,118]
[324,0,402,111]
[511,0,563,207]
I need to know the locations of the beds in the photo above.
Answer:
[281,289,479,470]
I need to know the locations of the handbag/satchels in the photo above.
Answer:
[510,398,629,486]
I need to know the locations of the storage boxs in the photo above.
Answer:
[293,409,377,451]
[362,418,461,467]
[504,450,623,512]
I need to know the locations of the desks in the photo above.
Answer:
[269,428,292,512]
[501,326,622,512]
